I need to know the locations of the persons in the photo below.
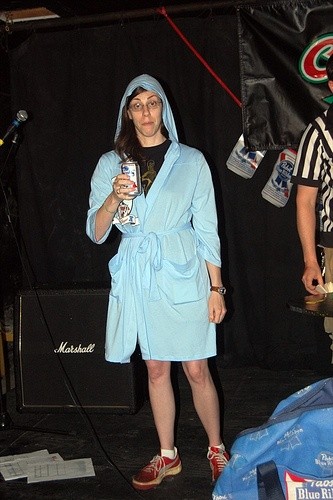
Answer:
[290,54,333,380]
[86,74,231,490]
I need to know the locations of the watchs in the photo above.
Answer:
[210,286,226,294]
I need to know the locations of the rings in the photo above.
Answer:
[116,188,120,194]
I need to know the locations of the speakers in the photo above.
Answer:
[14,289,138,413]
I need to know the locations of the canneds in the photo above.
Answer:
[226,133,268,179]
[121,161,142,196]
[261,148,298,208]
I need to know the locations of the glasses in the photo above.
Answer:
[128,99,162,112]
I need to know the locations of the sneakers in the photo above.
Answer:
[131,448,183,491]
[206,447,231,488]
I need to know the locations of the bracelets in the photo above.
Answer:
[104,202,114,214]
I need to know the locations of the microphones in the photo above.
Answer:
[0,111,27,146]
